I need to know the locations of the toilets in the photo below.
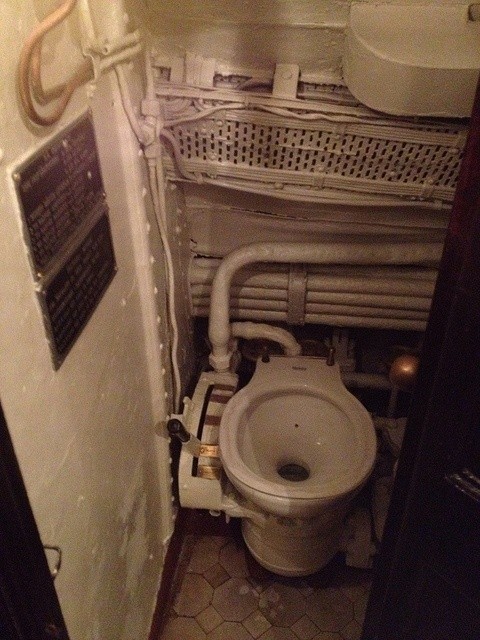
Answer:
[176,341,378,578]
